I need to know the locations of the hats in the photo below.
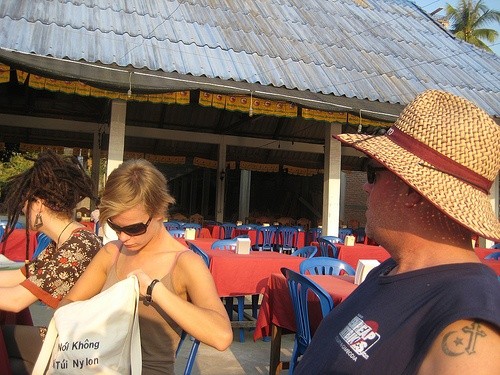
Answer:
[332,89,500,244]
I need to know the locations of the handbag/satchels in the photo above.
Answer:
[32,274,142,375]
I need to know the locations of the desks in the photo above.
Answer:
[200,248,311,344]
[252,273,360,375]
[172,237,233,250]
[235,228,306,258]
[310,242,392,276]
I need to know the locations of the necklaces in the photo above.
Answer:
[56,220,74,245]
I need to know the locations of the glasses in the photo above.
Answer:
[107,214,154,236]
[366,162,389,184]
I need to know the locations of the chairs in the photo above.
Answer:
[0,212,500,375]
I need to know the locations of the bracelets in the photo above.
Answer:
[146,279,160,303]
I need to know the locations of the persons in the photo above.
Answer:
[0,153,105,375]
[53,159,232,375]
[90,200,100,224]
[291,91,500,375]
